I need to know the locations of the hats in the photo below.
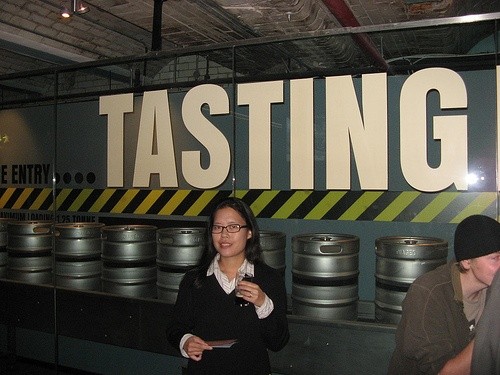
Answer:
[454,214,500,261]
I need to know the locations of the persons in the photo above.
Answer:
[386,213,500,375]
[167,197,290,375]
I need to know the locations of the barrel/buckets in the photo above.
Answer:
[0,218,15,266]
[157,228,207,290]
[7,221,54,270]
[258,229,286,280]
[51,223,102,276]
[291,233,360,320]
[102,225,158,283]
[374,236,448,322]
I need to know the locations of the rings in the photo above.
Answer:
[248,292,253,298]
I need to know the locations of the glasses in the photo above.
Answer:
[208,224,248,234]
